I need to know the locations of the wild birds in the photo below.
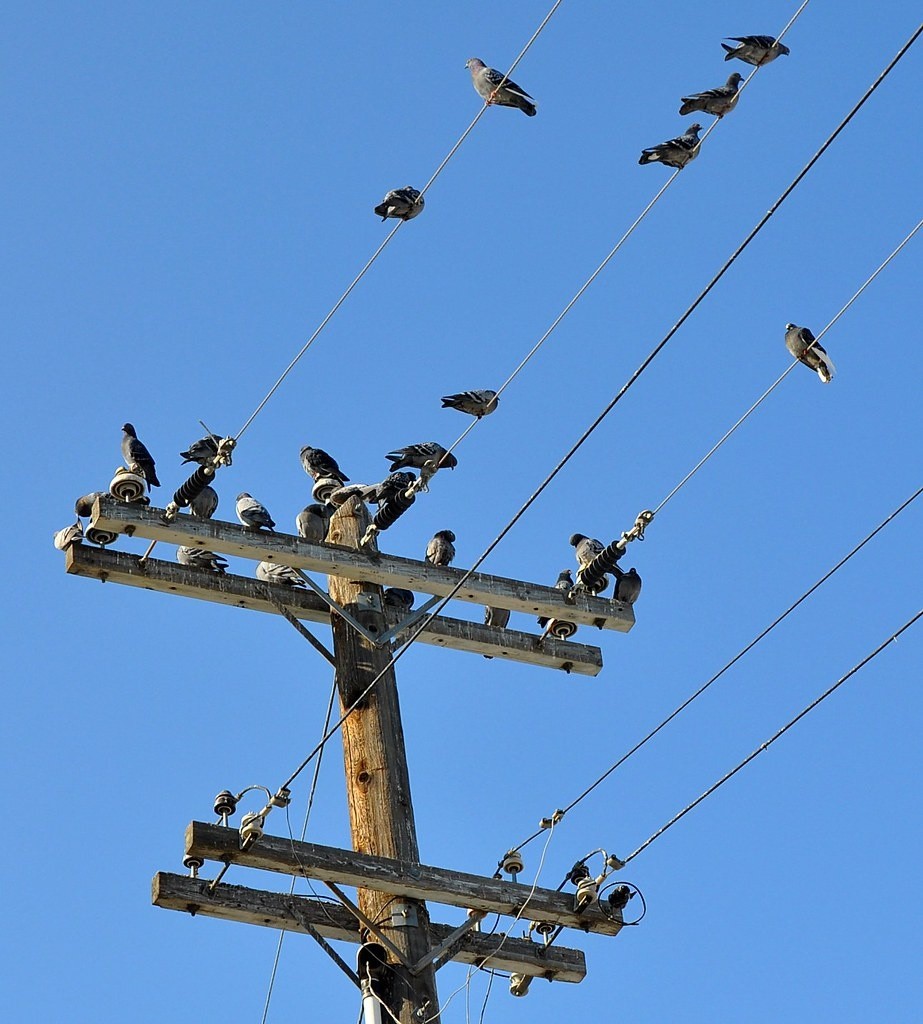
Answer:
[438,387,502,417]
[481,604,510,661]
[680,72,746,119]
[462,57,538,118]
[719,33,793,66]
[236,439,458,591]
[637,125,703,172]
[374,185,427,222]
[783,320,838,384]
[535,530,643,632]
[424,529,459,566]
[49,422,238,570]
